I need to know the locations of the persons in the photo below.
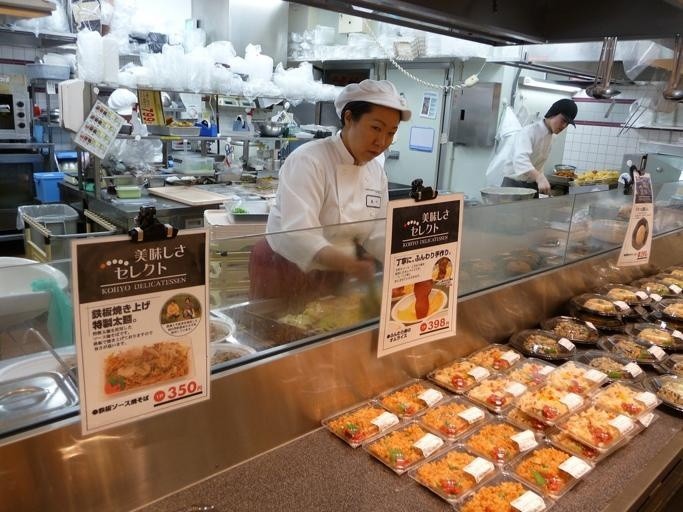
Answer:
[100,88,154,171]
[244,107,258,133]
[483,97,577,194]
[247,80,413,303]
[165,300,181,322]
[182,297,197,319]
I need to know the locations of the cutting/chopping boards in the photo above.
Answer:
[147,184,230,209]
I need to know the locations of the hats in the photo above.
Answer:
[334,79,411,121]
[545,99,578,128]
[108,88,139,117]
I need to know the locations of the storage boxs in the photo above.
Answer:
[33,171,66,202]
[17,205,78,246]
[171,155,215,174]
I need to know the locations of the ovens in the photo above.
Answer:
[0,151,45,258]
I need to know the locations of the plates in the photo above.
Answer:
[100,337,193,397]
[389,288,448,324]
[208,320,256,365]
[388,320,405,332]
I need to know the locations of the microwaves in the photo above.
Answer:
[0,90,28,141]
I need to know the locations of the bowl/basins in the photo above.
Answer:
[479,185,541,205]
[255,121,284,136]
[0,255,69,332]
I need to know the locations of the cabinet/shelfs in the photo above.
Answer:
[82,77,352,233]
